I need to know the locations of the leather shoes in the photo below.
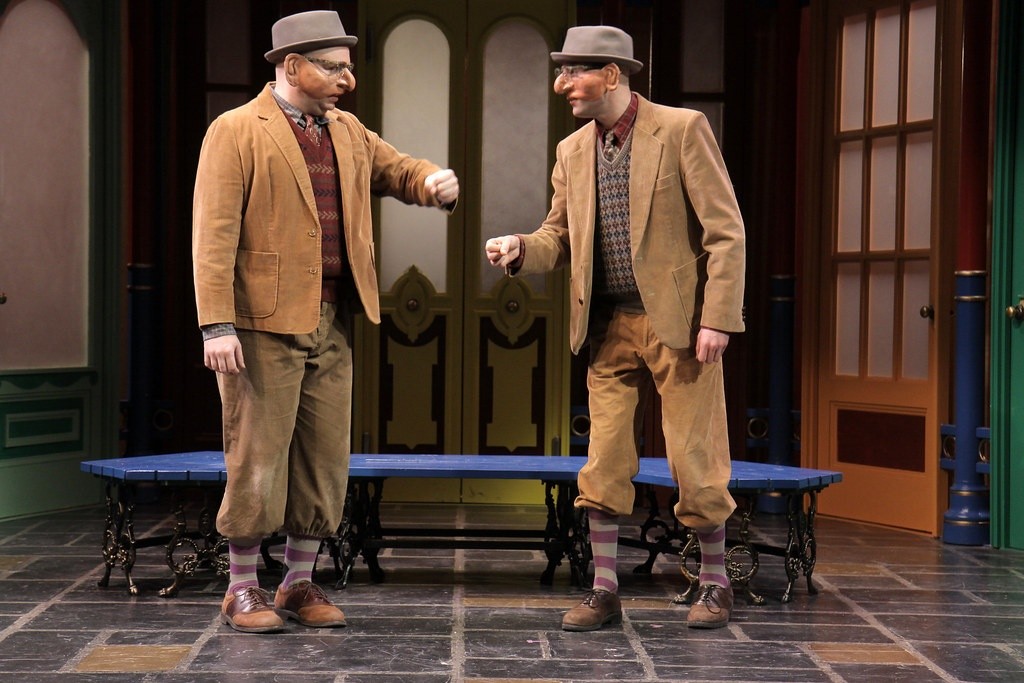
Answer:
[562,589,622,631]
[273,584,346,626]
[221,588,284,634]
[687,585,733,628]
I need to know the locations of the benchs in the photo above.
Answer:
[76,450,846,605]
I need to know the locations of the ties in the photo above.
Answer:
[303,114,320,147]
[603,131,620,162]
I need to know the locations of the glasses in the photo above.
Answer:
[305,56,354,75]
[555,66,602,81]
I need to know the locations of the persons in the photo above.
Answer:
[486,24,747,633]
[190,10,461,634]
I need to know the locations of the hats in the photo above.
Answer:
[264,10,358,64]
[549,25,643,76]
